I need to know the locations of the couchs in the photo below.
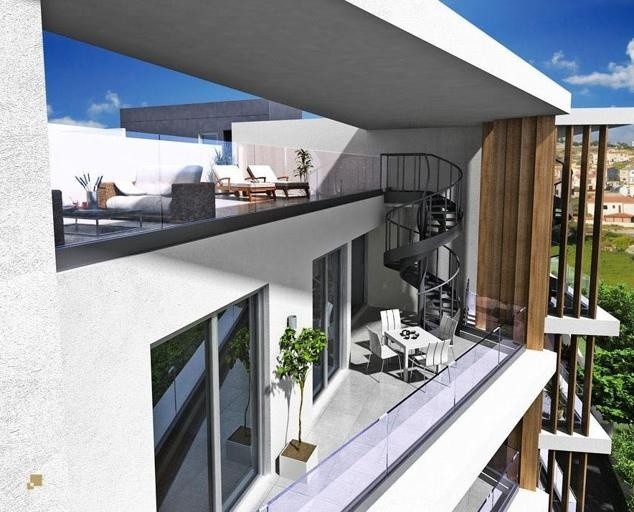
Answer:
[99,181,215,224]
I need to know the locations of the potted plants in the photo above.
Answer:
[223,327,251,469]
[273,327,329,482]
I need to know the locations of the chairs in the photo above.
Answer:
[380,308,401,369]
[410,340,452,393]
[433,314,458,367]
[366,326,402,380]
[211,163,277,203]
[243,165,311,200]
